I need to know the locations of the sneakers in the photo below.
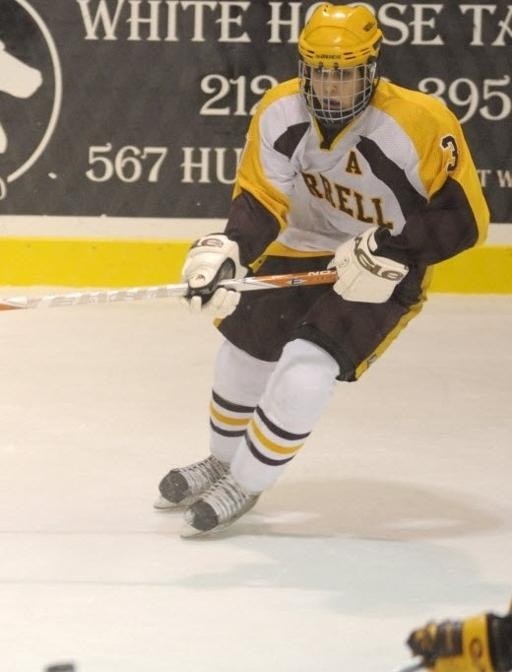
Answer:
[158,453,233,504]
[180,470,260,534]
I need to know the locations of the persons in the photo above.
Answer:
[394,604,512,672]
[153,1,491,536]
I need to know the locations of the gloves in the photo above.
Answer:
[182,231,250,327]
[324,223,411,307]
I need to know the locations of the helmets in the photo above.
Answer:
[297,2,386,72]
